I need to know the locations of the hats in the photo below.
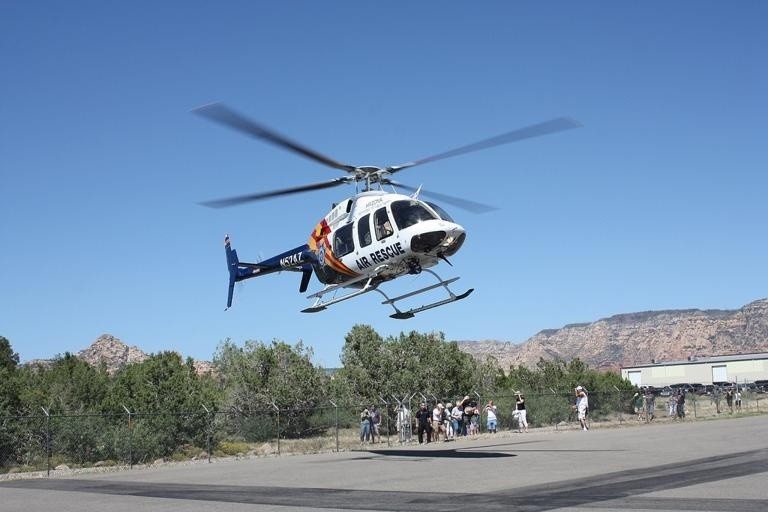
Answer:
[514,390,524,396]
[437,403,445,409]
[464,407,473,415]
[575,386,582,390]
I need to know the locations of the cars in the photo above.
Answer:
[639,380,768,396]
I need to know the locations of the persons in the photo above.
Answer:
[713,384,741,413]
[415,396,497,445]
[668,390,685,420]
[514,391,528,433]
[360,405,382,445]
[572,386,588,431]
[634,390,656,421]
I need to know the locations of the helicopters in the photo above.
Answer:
[186,97,583,319]
